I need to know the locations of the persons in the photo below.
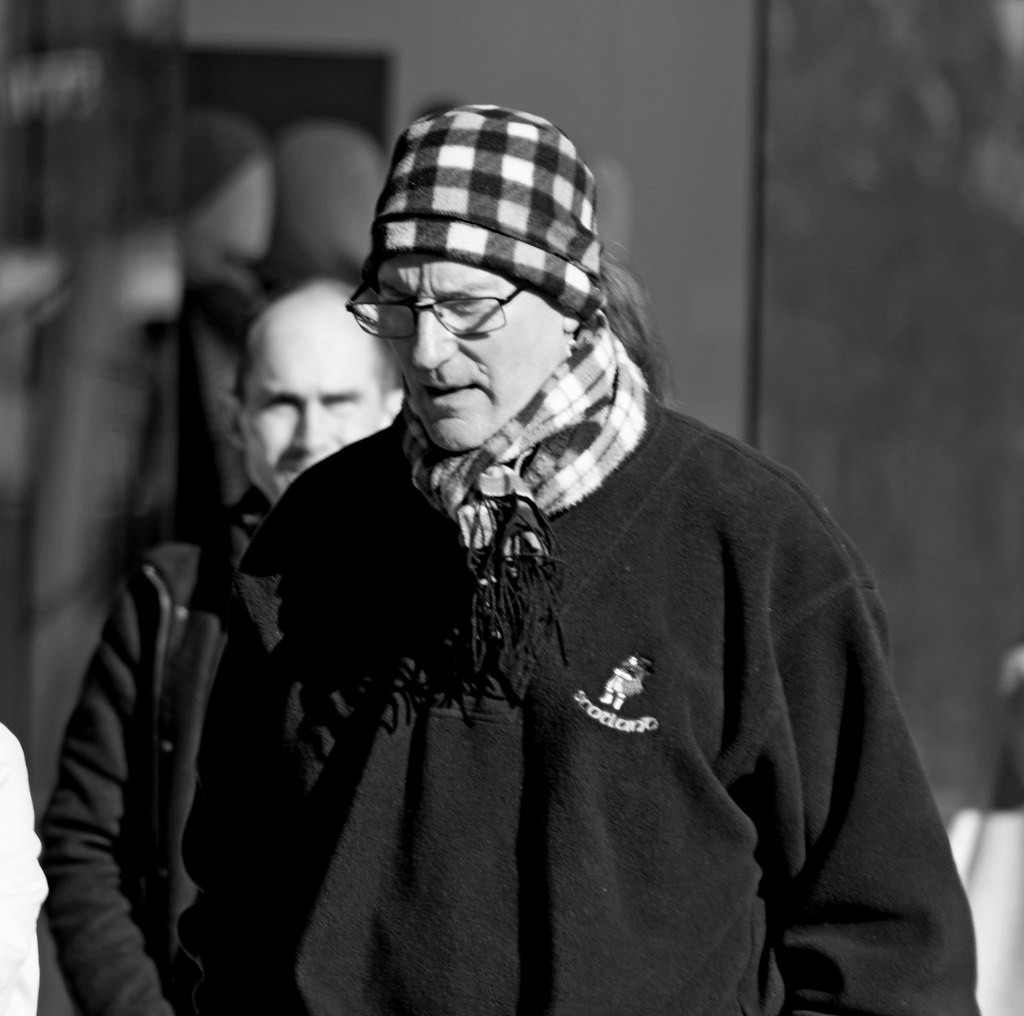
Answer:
[178,103,980,1016]
[38,278,403,1015]
[2,119,389,1014]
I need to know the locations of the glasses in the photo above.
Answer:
[345,264,520,338]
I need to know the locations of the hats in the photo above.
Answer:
[372,102,603,322]
